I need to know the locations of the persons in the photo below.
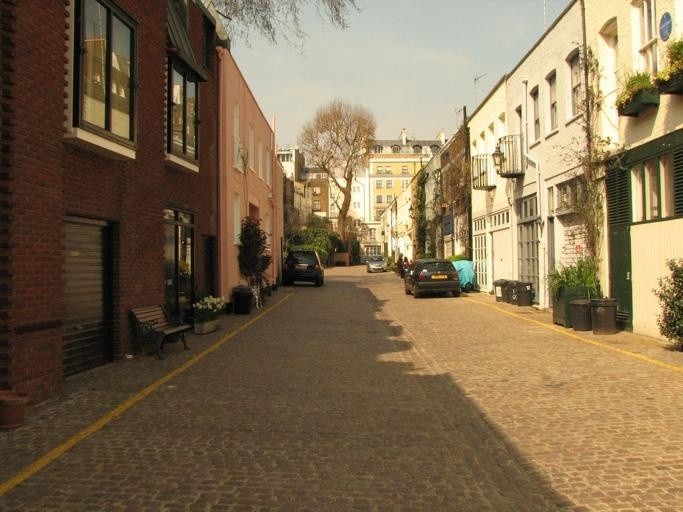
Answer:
[403,256,409,269]
[395,255,404,279]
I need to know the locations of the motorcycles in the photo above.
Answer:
[394,254,408,278]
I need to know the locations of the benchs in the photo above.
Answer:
[128,304,193,360]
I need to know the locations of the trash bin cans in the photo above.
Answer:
[570,298,618,335]
[232,286,253,315]
[493,279,532,306]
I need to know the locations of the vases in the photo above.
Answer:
[0,390,30,430]
[194,319,222,333]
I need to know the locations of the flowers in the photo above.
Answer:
[193,295,225,323]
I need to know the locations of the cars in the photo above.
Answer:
[283,251,323,287]
[403,259,460,298]
[365,255,386,273]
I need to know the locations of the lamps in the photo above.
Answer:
[492,142,510,176]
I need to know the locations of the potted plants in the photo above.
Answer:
[615,68,660,117]
[233,215,272,311]
[651,35,683,94]
[546,256,601,327]
